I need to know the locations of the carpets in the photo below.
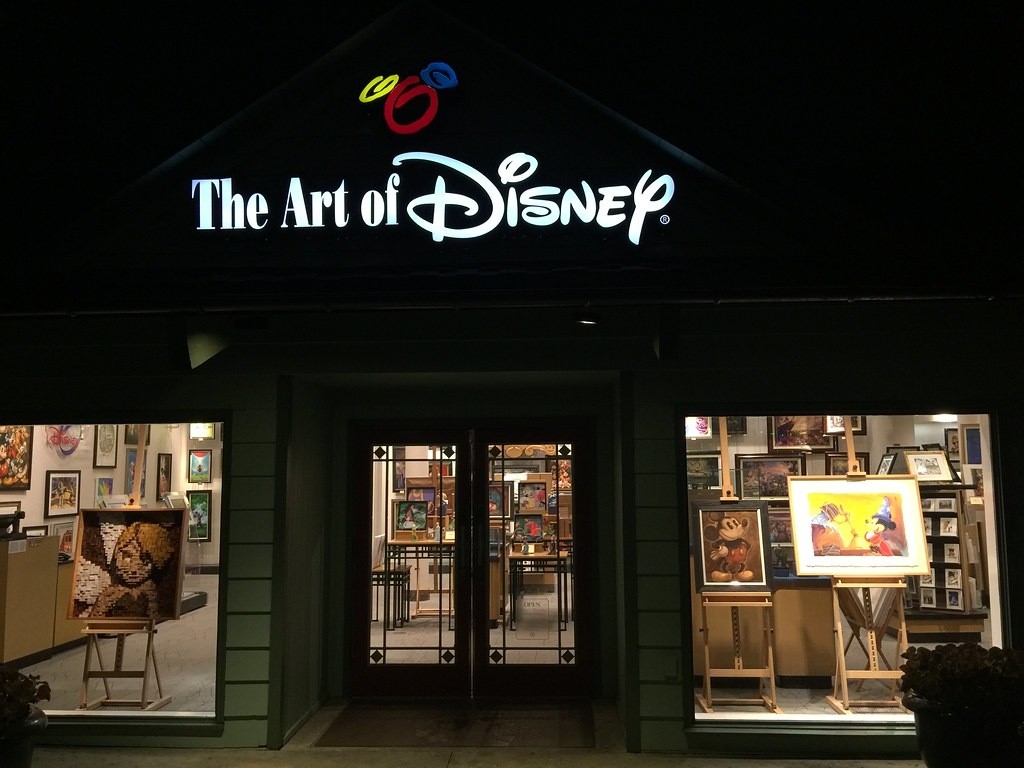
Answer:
[312,704,597,748]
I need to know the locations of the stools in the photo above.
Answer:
[374,565,412,631]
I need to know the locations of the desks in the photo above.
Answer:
[386,540,569,633]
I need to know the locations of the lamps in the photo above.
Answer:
[572,307,603,327]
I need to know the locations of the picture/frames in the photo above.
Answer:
[0,425,34,491]
[0,500,21,533]
[545,454,572,493]
[22,525,49,537]
[189,423,215,441]
[186,489,213,543]
[94,476,114,509]
[93,425,118,469]
[52,521,73,550]
[685,416,984,612]
[125,424,151,446]
[429,461,452,476]
[188,449,213,483]
[123,448,147,498]
[156,453,173,502]
[44,469,82,518]
[392,480,547,542]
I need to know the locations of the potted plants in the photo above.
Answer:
[899,642,1024,768]
[0,664,52,768]
